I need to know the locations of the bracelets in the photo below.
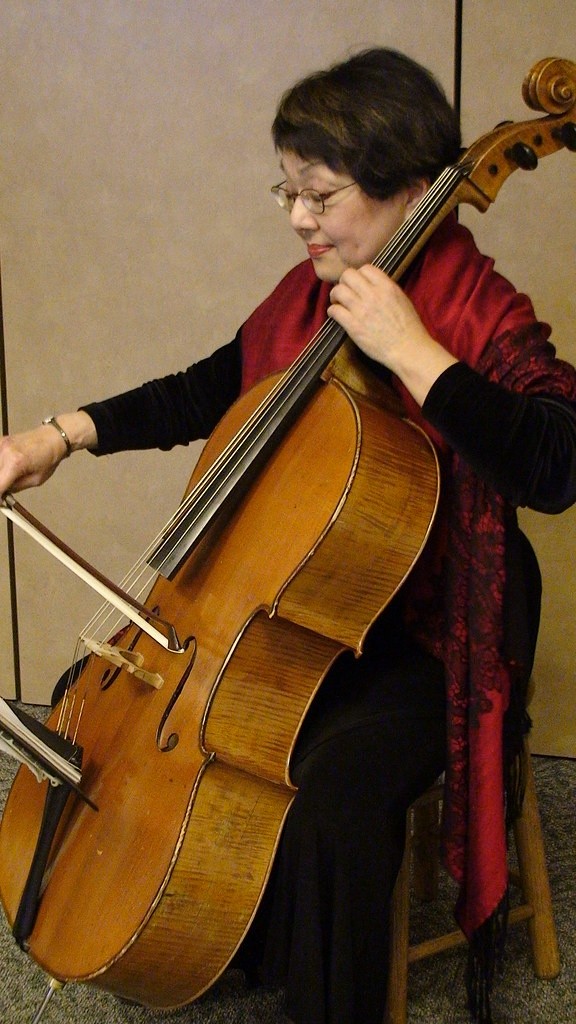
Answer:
[42,415,72,457]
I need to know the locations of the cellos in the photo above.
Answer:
[0,56,576,1024]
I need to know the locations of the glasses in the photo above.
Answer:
[269,180,357,215]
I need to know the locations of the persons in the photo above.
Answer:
[0,45,576,1023]
